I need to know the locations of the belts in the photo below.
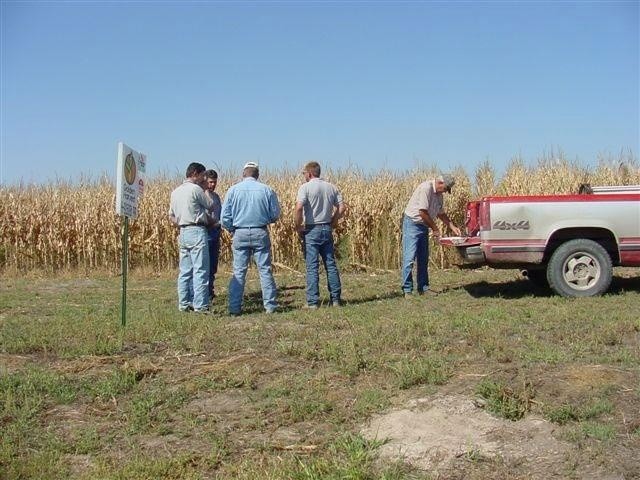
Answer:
[235,226,267,230]
[179,222,204,228]
[305,222,331,228]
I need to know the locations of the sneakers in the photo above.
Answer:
[331,300,344,308]
[301,302,319,310]
[420,289,440,297]
[403,291,417,301]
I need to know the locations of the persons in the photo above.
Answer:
[169,162,216,315]
[294,160,346,309]
[186,167,222,300]
[400,173,461,300]
[220,162,281,315]
[578,184,594,194]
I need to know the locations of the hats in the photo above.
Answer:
[440,173,455,194]
[243,160,259,171]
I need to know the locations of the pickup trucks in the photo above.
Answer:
[437,184,640,298]
